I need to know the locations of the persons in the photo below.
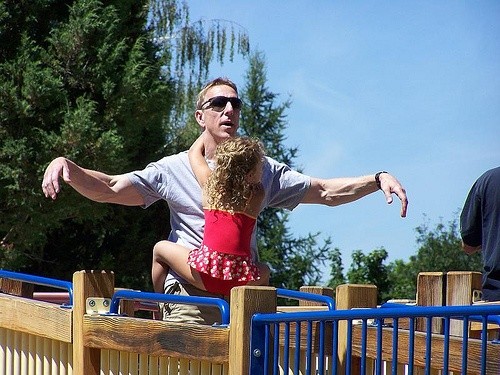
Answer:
[459,166,500,343]
[41,77,407,326]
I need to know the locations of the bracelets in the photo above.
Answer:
[375,171,388,190]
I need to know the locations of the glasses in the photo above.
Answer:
[200,96,242,113]
